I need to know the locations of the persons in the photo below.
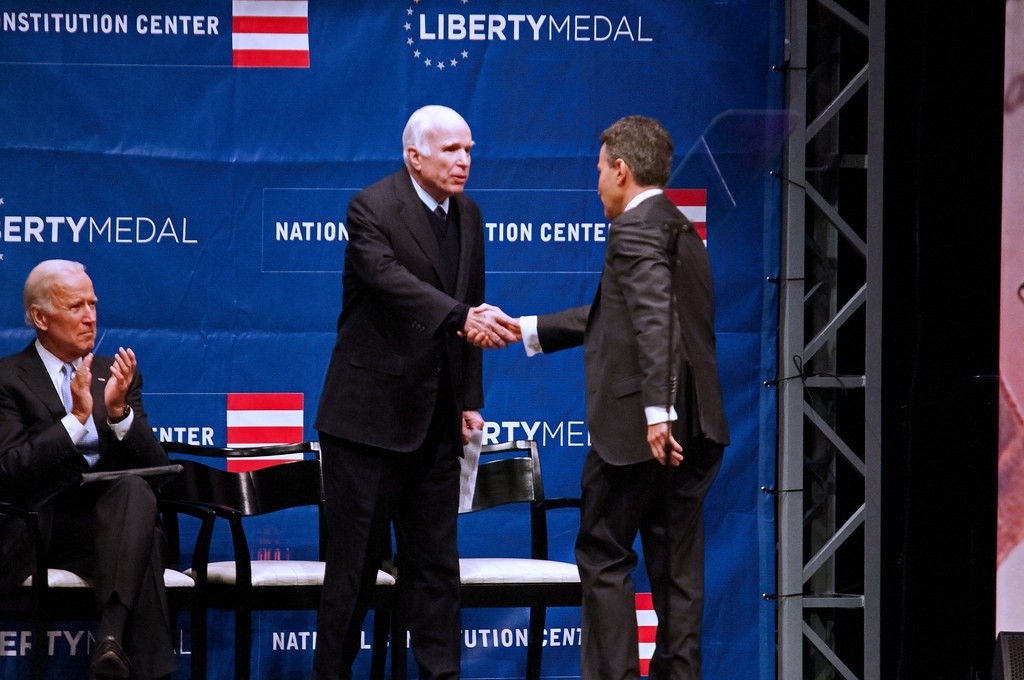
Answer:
[0,259,179,680]
[313,105,521,680]
[468,114,732,680]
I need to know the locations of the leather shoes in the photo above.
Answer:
[84,636,129,677]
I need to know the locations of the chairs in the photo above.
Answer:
[165,441,390,680]
[15,496,215,680]
[391,442,587,680]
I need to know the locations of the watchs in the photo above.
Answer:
[108,404,131,423]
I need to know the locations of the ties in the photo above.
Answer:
[59,364,102,469]
[434,208,450,245]
